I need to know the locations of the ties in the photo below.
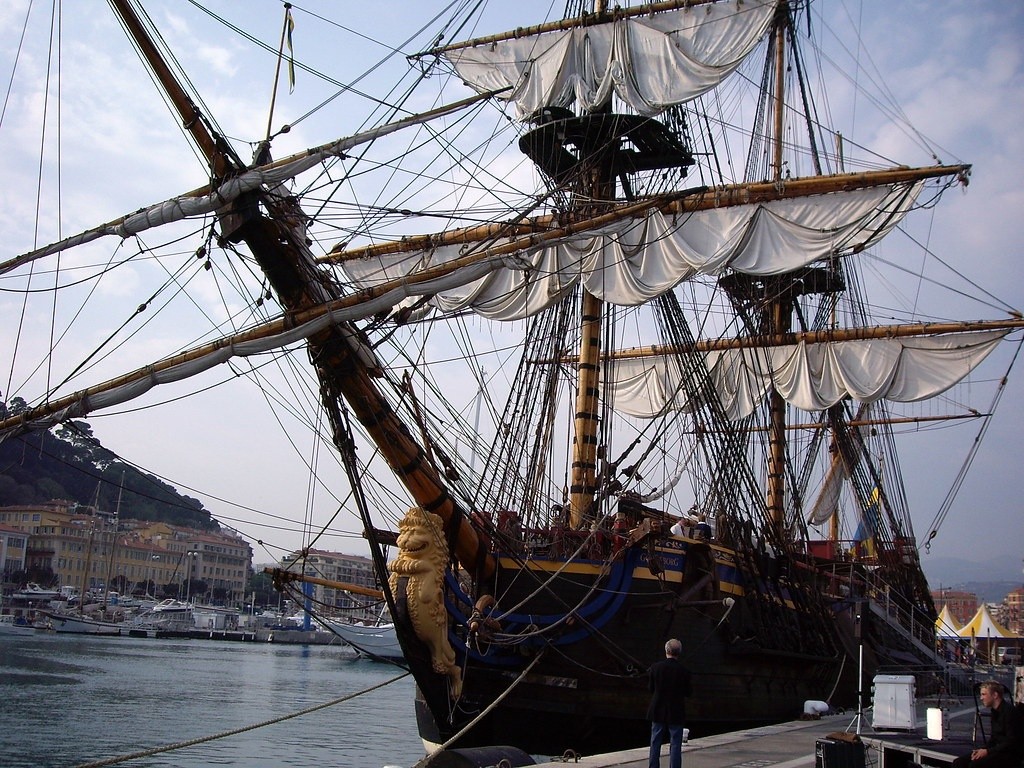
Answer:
[681,527,685,537]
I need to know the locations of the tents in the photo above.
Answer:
[933,603,1024,664]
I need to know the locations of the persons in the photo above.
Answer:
[468,505,853,564]
[931,638,1008,667]
[646,639,694,768]
[951,676,1024,768]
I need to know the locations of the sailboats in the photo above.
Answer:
[0,0,1024,768]
